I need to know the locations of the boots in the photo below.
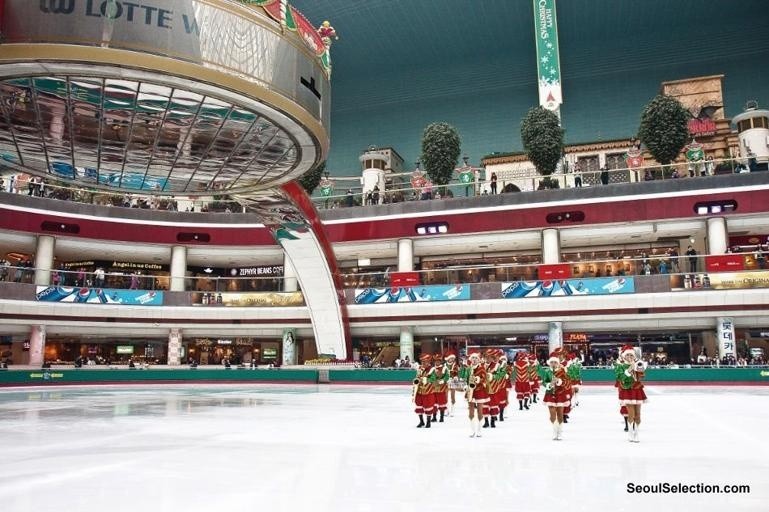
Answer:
[417,394,642,443]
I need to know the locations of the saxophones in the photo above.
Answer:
[411,366,424,404]
[467,364,476,403]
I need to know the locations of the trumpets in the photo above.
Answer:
[629,357,643,369]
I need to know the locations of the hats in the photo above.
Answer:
[418,344,637,362]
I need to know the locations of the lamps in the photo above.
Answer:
[693,199,740,215]
[415,219,448,236]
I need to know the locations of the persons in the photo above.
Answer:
[581,244,701,276]
[345,186,443,212]
[412,353,437,428]
[573,162,583,188]
[466,349,492,437]
[578,347,769,368]
[619,345,648,442]
[543,351,571,440]
[428,344,643,433]
[1,172,254,215]
[359,349,421,369]
[0,347,283,372]
[600,163,609,186]
[489,172,499,194]
[672,149,760,177]
[1,255,200,291]
